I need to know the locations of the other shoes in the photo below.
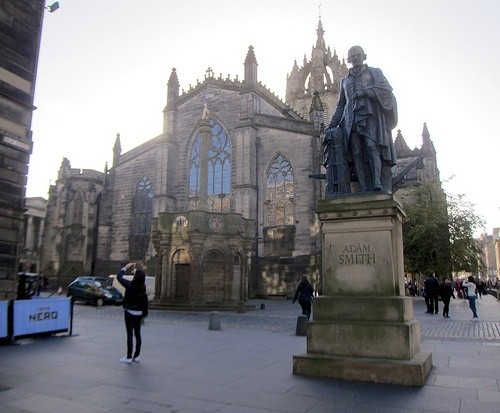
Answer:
[132,357,139,362]
[119,356,132,363]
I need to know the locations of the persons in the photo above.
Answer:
[424,276,435,314]
[116,262,149,363]
[292,276,314,321]
[327,46,398,192]
[428,273,439,314]
[438,278,455,318]
[404,272,500,302]
[462,276,479,321]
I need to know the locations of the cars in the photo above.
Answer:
[66,277,123,307]
